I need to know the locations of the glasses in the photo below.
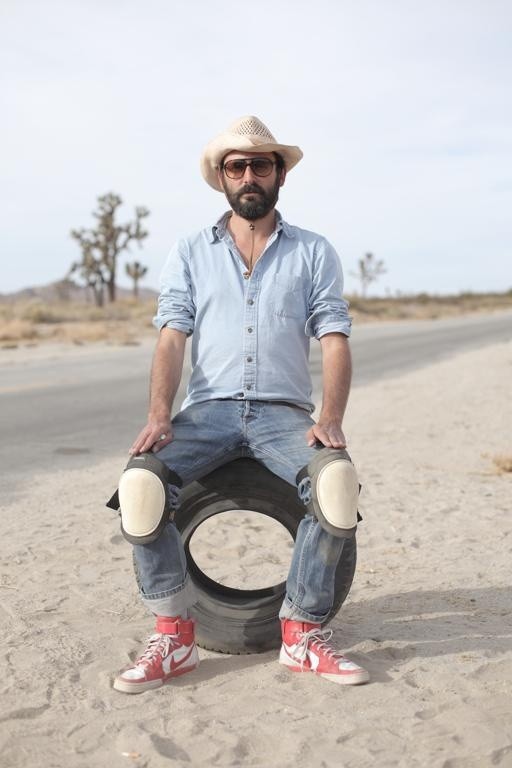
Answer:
[218,156,279,181]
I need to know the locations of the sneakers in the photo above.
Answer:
[277,615,372,688]
[111,612,202,694]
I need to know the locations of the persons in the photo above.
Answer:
[109,112,372,694]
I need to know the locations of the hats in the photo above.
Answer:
[199,114,305,193]
